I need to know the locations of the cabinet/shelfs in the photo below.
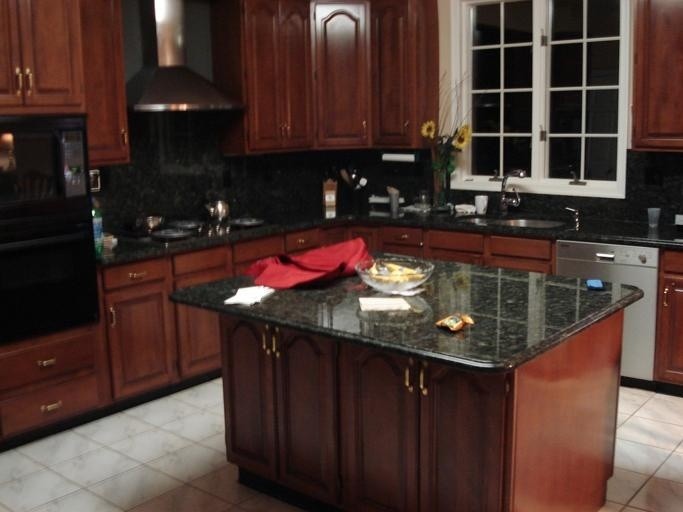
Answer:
[284,223,342,258]
[629,1,681,150]
[2,0,85,116]
[0,319,109,444]
[211,1,309,158]
[104,258,180,406]
[77,0,129,168]
[343,225,379,254]
[170,239,231,382]
[374,4,438,147]
[163,250,644,512]
[480,233,553,273]
[424,230,480,261]
[306,1,375,150]
[653,244,682,387]
[380,228,424,257]
[229,231,285,278]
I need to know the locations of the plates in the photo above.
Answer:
[151,215,265,240]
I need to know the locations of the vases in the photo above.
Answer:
[434,172,447,208]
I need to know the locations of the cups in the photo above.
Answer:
[419,189,431,210]
[475,195,488,214]
[389,191,400,212]
[647,207,661,228]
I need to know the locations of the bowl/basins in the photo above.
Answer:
[355,293,433,328]
[355,257,436,293]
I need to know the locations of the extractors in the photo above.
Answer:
[125,0,245,114]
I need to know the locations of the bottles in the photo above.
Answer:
[90,206,103,255]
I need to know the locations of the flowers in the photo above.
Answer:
[420,115,470,176]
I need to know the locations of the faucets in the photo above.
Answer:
[499,168,527,211]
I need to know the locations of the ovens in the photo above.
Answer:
[0,108,105,344]
[556,239,659,384]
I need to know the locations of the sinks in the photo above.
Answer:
[459,217,565,231]
